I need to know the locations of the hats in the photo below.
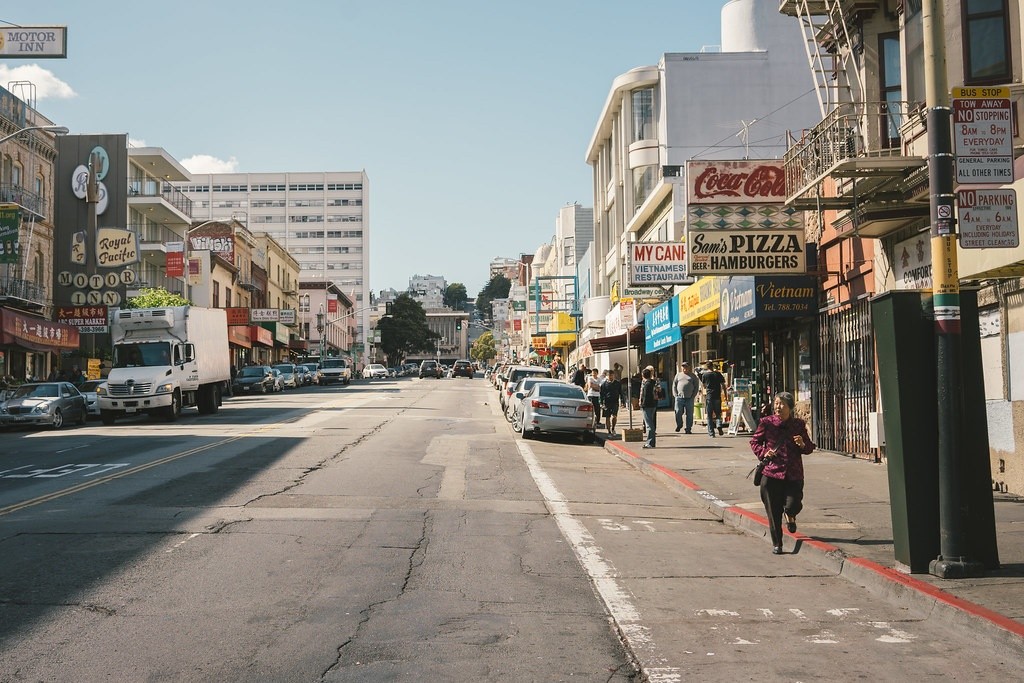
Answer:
[682,362,691,367]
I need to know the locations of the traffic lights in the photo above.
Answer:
[456,318,462,330]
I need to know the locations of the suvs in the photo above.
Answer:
[316,358,353,385]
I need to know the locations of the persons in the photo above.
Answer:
[639,366,661,448]
[531,358,565,379]
[702,362,727,437]
[46,366,85,384]
[672,363,699,433]
[572,362,625,436]
[750,392,813,553]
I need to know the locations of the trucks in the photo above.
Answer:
[97,305,231,424]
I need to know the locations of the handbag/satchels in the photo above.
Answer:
[754,463,765,486]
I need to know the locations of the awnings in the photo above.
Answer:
[589,333,643,352]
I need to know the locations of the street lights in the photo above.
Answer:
[324,278,358,356]
[493,256,531,367]
[184,217,234,302]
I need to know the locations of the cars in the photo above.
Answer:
[273,363,322,389]
[233,365,276,394]
[504,377,568,422]
[387,363,419,378]
[510,382,597,445]
[0,381,90,431]
[419,359,440,380]
[485,360,554,412]
[361,363,390,379]
[270,368,285,392]
[437,361,479,378]
[78,378,109,421]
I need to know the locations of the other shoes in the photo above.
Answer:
[642,444,655,448]
[784,512,796,533]
[686,431,692,434]
[710,435,714,438]
[675,425,683,432]
[773,547,782,554]
[612,430,616,436]
[608,433,612,437]
[718,427,723,435]
